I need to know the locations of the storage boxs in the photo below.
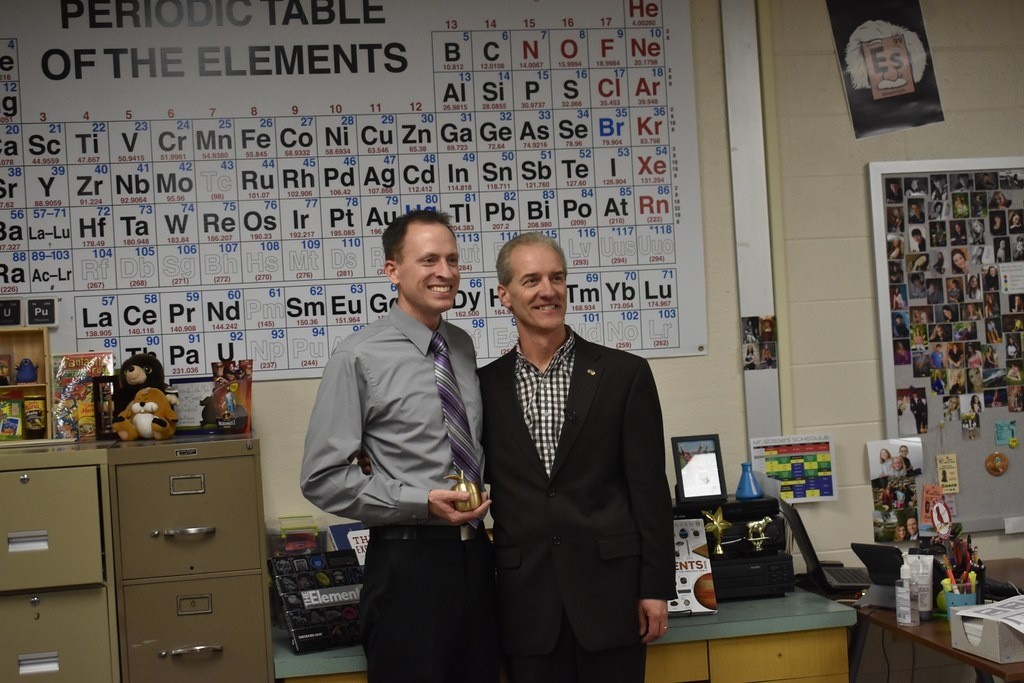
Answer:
[951,605,1024,665]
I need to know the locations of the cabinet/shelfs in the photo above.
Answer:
[106,424,275,683]
[0,439,121,683]
[0,327,52,440]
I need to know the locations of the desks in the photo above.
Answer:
[857,607,1024,683]
[274,587,857,683]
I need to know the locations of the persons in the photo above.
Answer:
[476,233,677,682]
[871,444,919,542]
[884,173,1024,440]
[300,209,492,682]
[743,316,776,370]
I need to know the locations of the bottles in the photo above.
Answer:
[895,565,920,626]
[735,463,762,498]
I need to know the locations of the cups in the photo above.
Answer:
[941,579,978,621]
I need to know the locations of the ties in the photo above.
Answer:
[432,328,487,530]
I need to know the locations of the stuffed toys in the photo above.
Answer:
[15,358,38,382]
[111,353,179,440]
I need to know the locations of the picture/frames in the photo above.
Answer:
[671,433,727,503]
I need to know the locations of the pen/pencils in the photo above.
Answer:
[942,534,979,594]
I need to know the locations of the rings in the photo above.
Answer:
[664,626,667,629]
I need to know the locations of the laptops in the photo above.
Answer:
[780,497,872,591]
